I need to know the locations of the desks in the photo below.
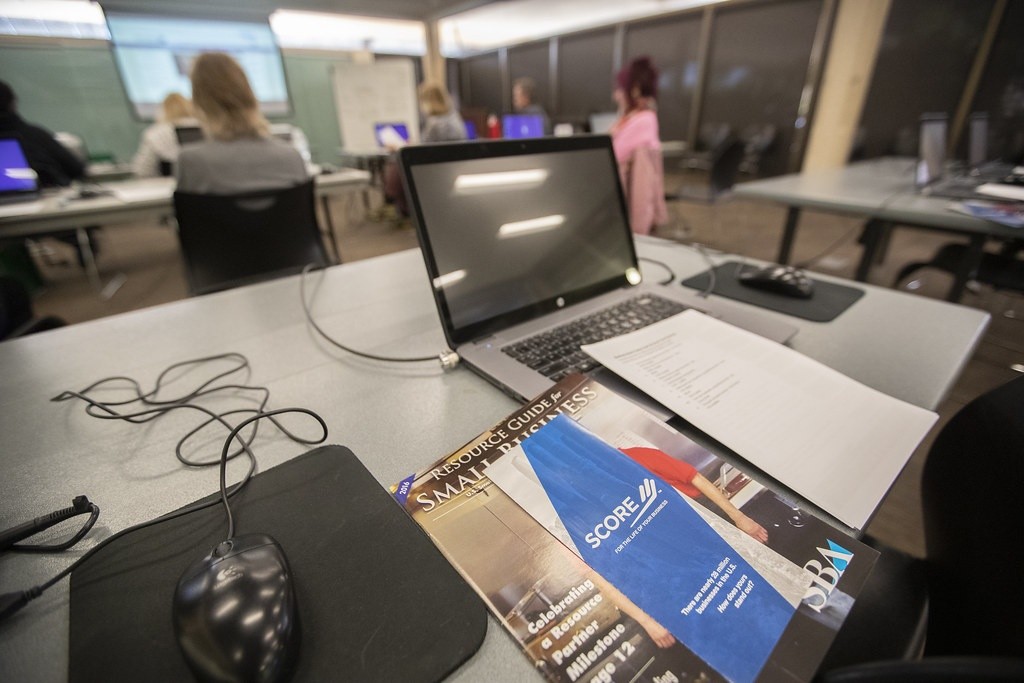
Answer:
[0,234,990,683]
[0,163,371,296]
[730,155,1024,303]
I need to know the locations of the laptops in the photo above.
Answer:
[916,113,1023,204]
[0,133,44,203]
[373,110,799,408]
[175,125,204,145]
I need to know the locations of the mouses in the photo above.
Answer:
[736,264,816,299]
[174,533,300,683]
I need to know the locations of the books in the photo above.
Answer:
[948,197,1024,229]
[390,372,880,682]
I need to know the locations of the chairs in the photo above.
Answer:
[807,373,1024,683]
[171,179,331,299]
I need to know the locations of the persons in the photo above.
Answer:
[512,77,553,136]
[368,84,467,228]
[0,81,99,263]
[560,447,768,648]
[137,51,308,193]
[611,57,667,236]
